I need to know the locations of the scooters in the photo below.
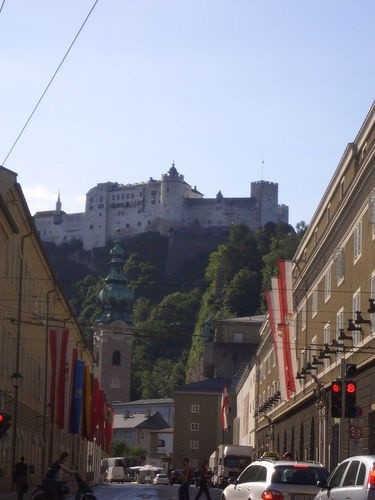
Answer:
[31,465,97,500]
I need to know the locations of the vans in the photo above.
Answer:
[105,457,165,485]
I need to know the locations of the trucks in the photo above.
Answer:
[209,444,254,489]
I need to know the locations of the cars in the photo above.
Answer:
[313,456,375,500]
[153,474,169,485]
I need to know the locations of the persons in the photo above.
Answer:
[44,451,70,481]
[194,462,215,500]
[178,457,193,500]
[13,455,28,500]
[168,470,178,485]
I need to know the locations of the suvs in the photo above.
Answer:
[221,458,330,500]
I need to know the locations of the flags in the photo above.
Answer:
[49,327,114,452]
[264,260,301,400]
[219,385,231,432]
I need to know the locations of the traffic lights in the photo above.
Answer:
[344,381,358,419]
[330,381,342,418]
[0,413,13,437]
